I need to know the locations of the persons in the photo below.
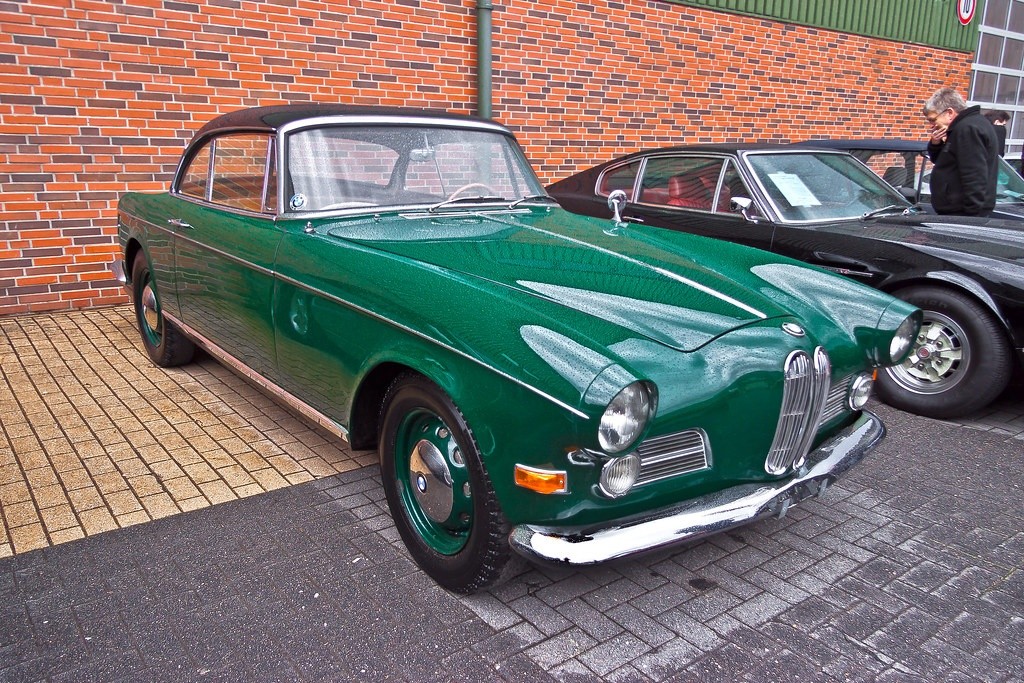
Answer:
[984,110,1010,157]
[923,87,999,220]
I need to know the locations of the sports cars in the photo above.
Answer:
[544,143,1024,420]
[117,103,924,594]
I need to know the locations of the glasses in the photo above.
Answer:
[930,108,948,125]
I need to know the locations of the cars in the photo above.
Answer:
[799,139,1024,221]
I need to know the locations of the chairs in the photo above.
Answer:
[665,175,725,213]
[883,166,907,186]
